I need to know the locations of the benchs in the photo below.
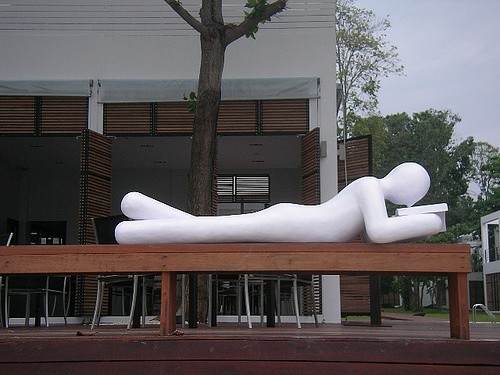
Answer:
[0,242,473,340]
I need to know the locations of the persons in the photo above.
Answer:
[115,160,442,243]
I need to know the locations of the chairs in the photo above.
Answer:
[0,232,71,328]
[91,215,319,329]
